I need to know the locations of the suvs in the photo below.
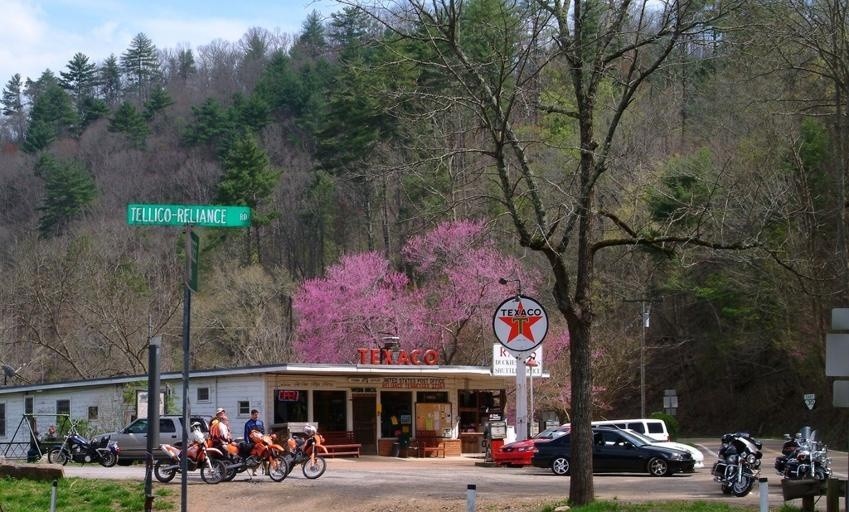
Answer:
[90,416,211,468]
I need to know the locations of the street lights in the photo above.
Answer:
[526,356,539,438]
[498,276,528,440]
[638,302,652,417]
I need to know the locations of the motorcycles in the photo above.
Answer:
[773,425,831,483]
[150,420,228,485]
[220,430,289,483]
[45,420,120,468]
[710,430,763,497]
[285,424,329,480]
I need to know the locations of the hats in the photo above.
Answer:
[216,408,225,416]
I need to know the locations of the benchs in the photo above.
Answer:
[317,430,362,460]
[414,430,448,458]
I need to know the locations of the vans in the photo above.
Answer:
[554,417,670,442]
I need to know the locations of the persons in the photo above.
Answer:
[208,407,233,445]
[46,425,57,441]
[243,409,270,475]
[26,431,46,460]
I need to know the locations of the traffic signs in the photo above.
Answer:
[127,201,252,228]
[182,226,201,296]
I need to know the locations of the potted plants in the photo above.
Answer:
[398,432,410,458]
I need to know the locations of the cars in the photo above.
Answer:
[531,426,695,477]
[495,424,571,465]
[604,428,705,470]
